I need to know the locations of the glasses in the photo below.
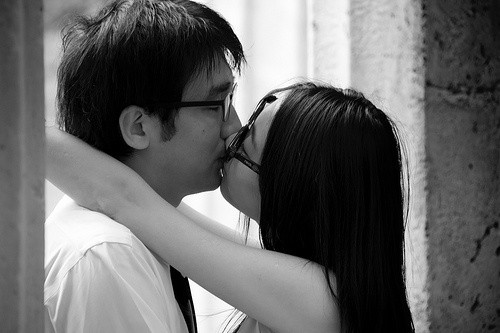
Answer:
[175,84,238,122]
[227,93,278,183]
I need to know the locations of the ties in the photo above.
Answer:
[170,268,199,333]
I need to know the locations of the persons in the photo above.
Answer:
[42,1,242,333]
[43,80,416,333]
